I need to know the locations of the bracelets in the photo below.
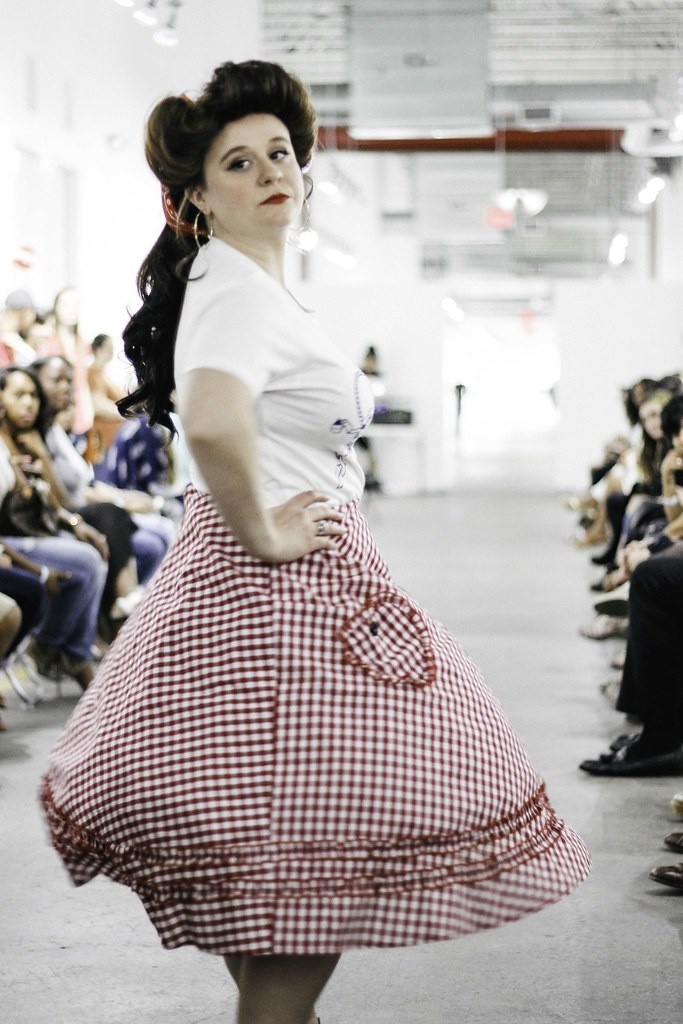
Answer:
[68,514,82,527]
[40,566,49,584]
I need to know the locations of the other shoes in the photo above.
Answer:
[600,649,626,702]
[590,581,605,591]
[70,657,93,690]
[592,554,620,573]
[664,832,683,853]
[4,635,84,706]
[579,731,683,778]
[579,580,631,638]
[648,862,683,888]
[670,793,683,820]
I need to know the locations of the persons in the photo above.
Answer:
[0,285,187,733]
[358,347,385,489]
[39,58,591,1024]
[564,376,683,896]
